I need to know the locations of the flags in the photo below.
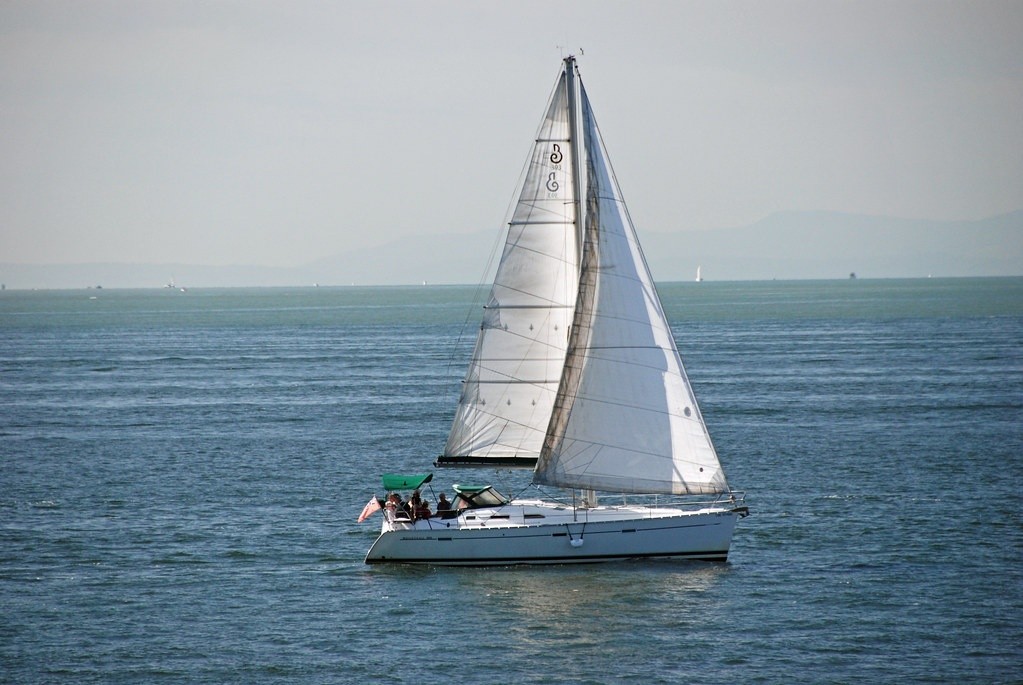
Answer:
[355,493,381,524]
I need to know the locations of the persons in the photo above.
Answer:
[380,489,434,527]
[431,493,451,518]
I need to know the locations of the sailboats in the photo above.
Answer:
[696,265,704,281]
[354,48,752,569]
